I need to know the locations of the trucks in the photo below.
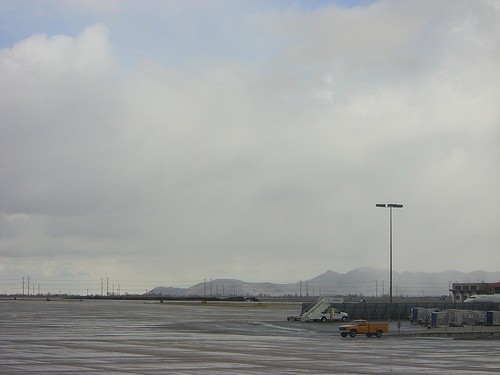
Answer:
[299,295,348,322]
[338,319,388,338]
